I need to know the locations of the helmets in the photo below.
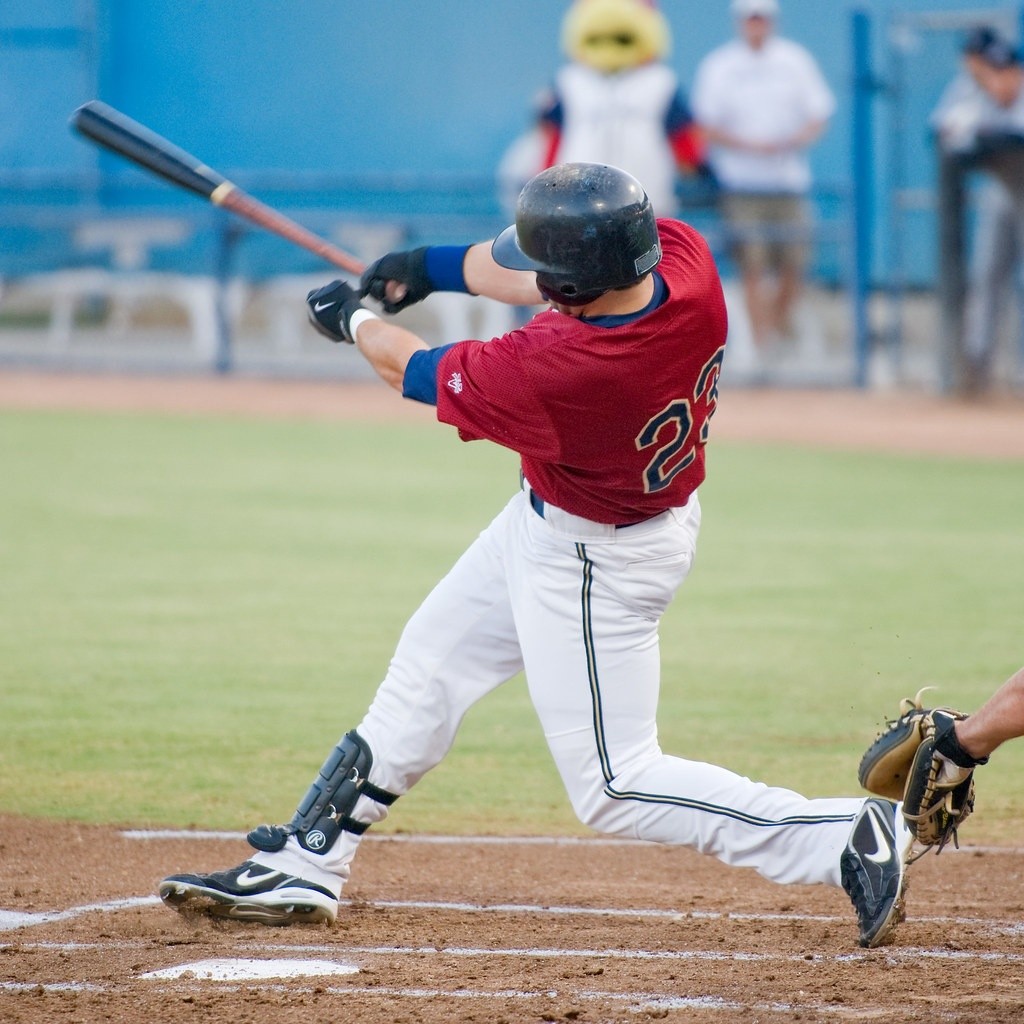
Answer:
[490,164,662,306]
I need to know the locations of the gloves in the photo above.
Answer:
[353,245,433,317]
[305,280,362,343]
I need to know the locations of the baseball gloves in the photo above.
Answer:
[853,701,998,851]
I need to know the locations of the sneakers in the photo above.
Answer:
[839,797,917,946]
[158,860,337,928]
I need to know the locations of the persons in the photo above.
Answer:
[487,0,1024,396]
[160,164,910,948]
[859,666,1024,866]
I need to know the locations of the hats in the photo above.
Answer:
[983,42,1018,67]
[728,0,775,20]
[957,25,994,54]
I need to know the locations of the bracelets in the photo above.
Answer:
[350,308,381,342]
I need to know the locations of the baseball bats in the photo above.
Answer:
[67,100,408,305]
